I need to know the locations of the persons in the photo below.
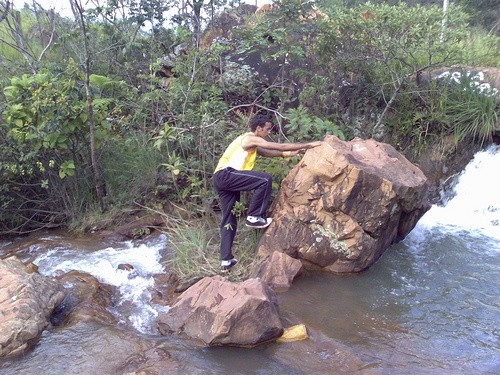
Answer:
[212,111,323,272]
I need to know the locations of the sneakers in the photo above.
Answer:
[220,259,237,273]
[246,217,272,229]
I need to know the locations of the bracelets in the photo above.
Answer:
[282,151,291,158]
